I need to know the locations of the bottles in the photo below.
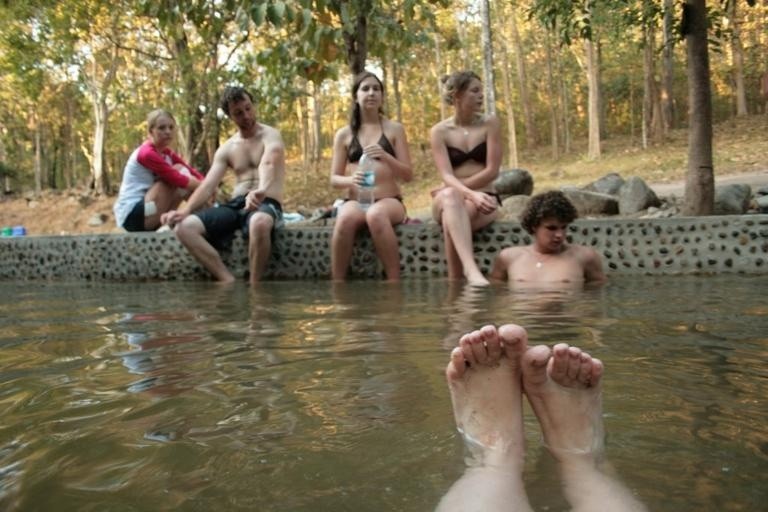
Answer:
[357,150,375,212]
[0,226,27,237]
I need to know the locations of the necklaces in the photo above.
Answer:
[455,121,477,138]
[532,253,554,269]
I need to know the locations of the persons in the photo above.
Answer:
[433,318,649,512]
[160,84,290,321]
[427,68,509,290]
[490,188,611,293]
[325,69,416,285]
[109,107,218,233]
